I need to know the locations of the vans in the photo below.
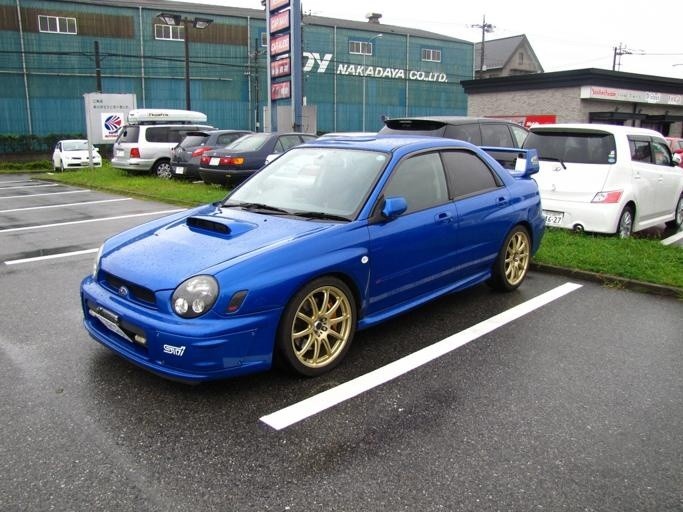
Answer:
[111,109,218,179]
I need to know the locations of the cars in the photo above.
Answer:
[170,129,319,187]
[79,136,545,386]
[52,139,103,173]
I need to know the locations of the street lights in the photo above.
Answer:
[361,34,381,128]
[157,13,212,111]
